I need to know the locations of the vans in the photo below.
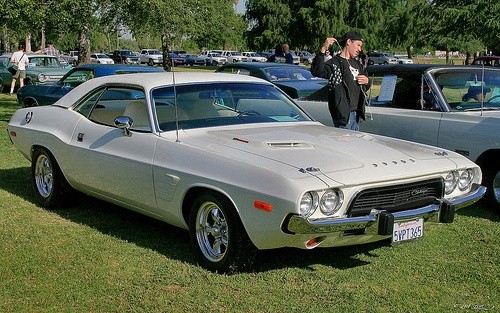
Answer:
[367,52,397,65]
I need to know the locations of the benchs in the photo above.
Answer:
[92,107,179,126]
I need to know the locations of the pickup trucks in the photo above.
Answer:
[137,49,164,66]
[63,51,79,66]
[106,50,141,65]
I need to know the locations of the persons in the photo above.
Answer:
[266,43,294,64]
[323,50,333,62]
[9,44,29,95]
[311,30,372,132]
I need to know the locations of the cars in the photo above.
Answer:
[89,54,114,65]
[172,50,273,67]
[57,56,69,65]
[393,55,414,64]
[168,53,183,66]
[289,49,315,64]
[214,61,329,101]
[24,54,88,89]
[470,57,500,68]
[17,64,182,118]
[8,71,485,274]
[0,56,22,92]
[235,63,499,217]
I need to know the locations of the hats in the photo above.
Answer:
[335,31,363,46]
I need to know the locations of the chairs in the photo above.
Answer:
[176,87,221,120]
[123,101,150,127]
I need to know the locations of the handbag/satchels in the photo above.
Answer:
[8,65,18,74]
